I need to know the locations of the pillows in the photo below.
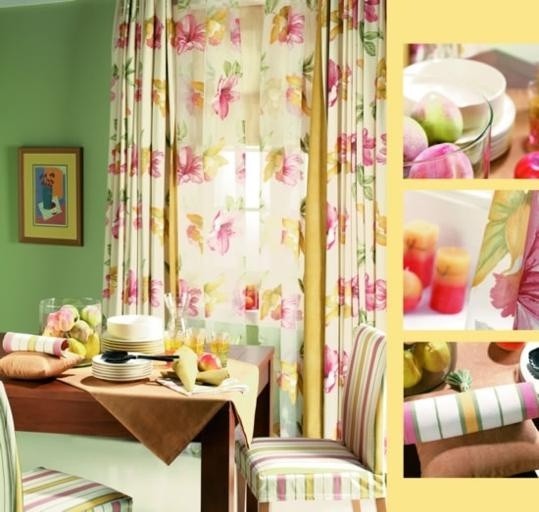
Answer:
[0,346,86,385]
[404,367,539,477]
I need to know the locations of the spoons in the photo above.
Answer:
[100,349,179,363]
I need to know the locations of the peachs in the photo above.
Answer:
[48,304,103,340]
[408,141,476,180]
[198,353,222,371]
[404,115,428,164]
[410,91,464,144]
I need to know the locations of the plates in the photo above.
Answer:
[91,352,152,381]
[405,342,457,397]
[453,93,518,165]
[100,336,166,365]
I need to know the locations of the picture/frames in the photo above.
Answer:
[15,142,85,248]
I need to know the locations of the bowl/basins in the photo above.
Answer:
[106,315,165,337]
[402,59,510,129]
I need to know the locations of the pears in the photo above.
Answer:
[404,349,423,389]
[85,330,100,358]
[65,333,87,357]
[416,342,450,373]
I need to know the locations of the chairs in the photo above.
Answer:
[0,381,137,510]
[232,320,395,512]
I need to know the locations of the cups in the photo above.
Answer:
[164,292,191,352]
[431,246,471,317]
[37,297,102,368]
[523,78,539,153]
[405,88,494,178]
[181,327,206,353]
[208,332,230,368]
[404,244,438,292]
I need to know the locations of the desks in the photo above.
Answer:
[0,342,284,511]
[460,47,539,178]
